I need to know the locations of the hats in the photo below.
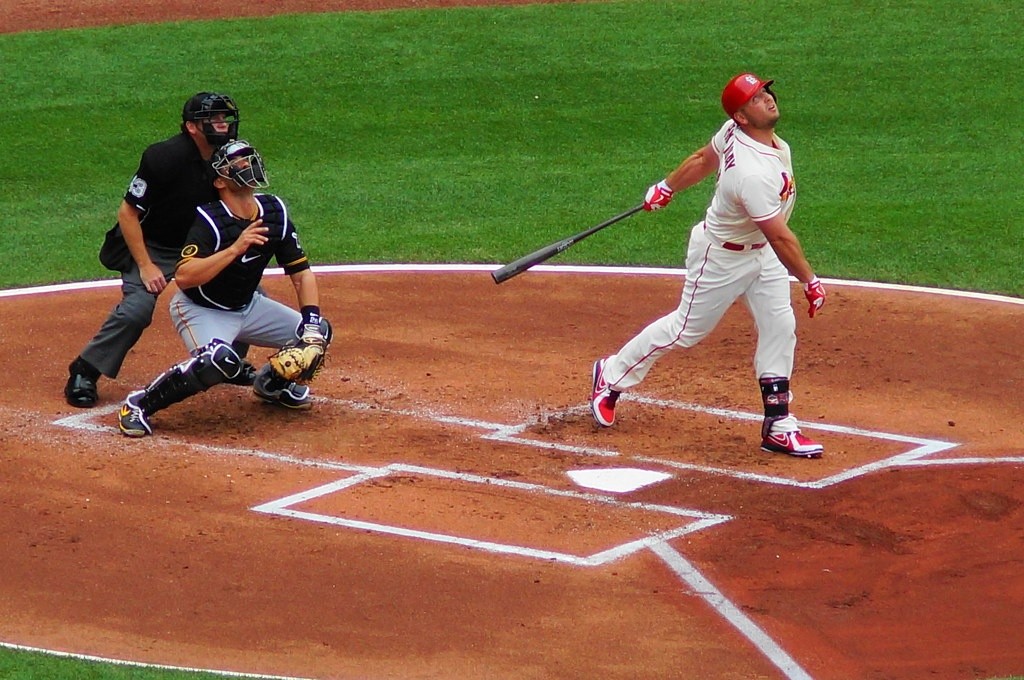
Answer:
[182,92,231,122]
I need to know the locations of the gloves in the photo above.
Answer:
[643,179,674,212]
[800,273,826,318]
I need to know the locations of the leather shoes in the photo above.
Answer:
[65,372,98,406]
[228,360,256,386]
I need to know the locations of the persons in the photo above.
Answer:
[65,90,240,408]
[118,139,333,436]
[591,73,826,458]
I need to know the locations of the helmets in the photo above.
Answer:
[721,73,777,125]
[209,139,255,182]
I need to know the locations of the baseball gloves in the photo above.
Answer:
[267,334,328,389]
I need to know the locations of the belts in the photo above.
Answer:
[703,222,765,251]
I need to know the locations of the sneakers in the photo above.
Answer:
[760,414,824,458]
[253,366,313,410]
[117,392,146,437]
[591,358,622,426]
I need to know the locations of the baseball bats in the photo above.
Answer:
[491,203,643,285]
